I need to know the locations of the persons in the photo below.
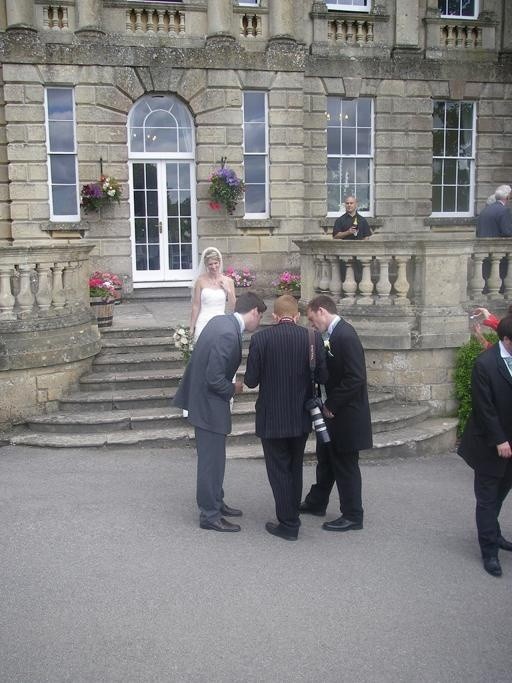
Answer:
[242,294,330,540]
[456,315,512,576]
[472,304,512,350]
[486,194,496,207]
[171,290,266,532]
[182,245,237,418]
[332,196,372,294]
[476,184,512,296]
[291,296,372,530]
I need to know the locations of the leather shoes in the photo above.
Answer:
[199,516,241,533]
[265,522,299,542]
[298,501,327,516]
[482,554,503,577]
[221,504,243,516]
[323,516,364,531]
[496,535,512,551]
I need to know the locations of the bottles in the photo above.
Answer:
[353,216,358,229]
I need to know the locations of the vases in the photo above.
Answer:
[227,282,252,301]
[275,287,303,302]
[89,295,115,327]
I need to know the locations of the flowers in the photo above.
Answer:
[271,268,302,290]
[224,264,257,287]
[79,174,124,215]
[173,323,193,364]
[207,167,247,217]
[86,269,124,304]
